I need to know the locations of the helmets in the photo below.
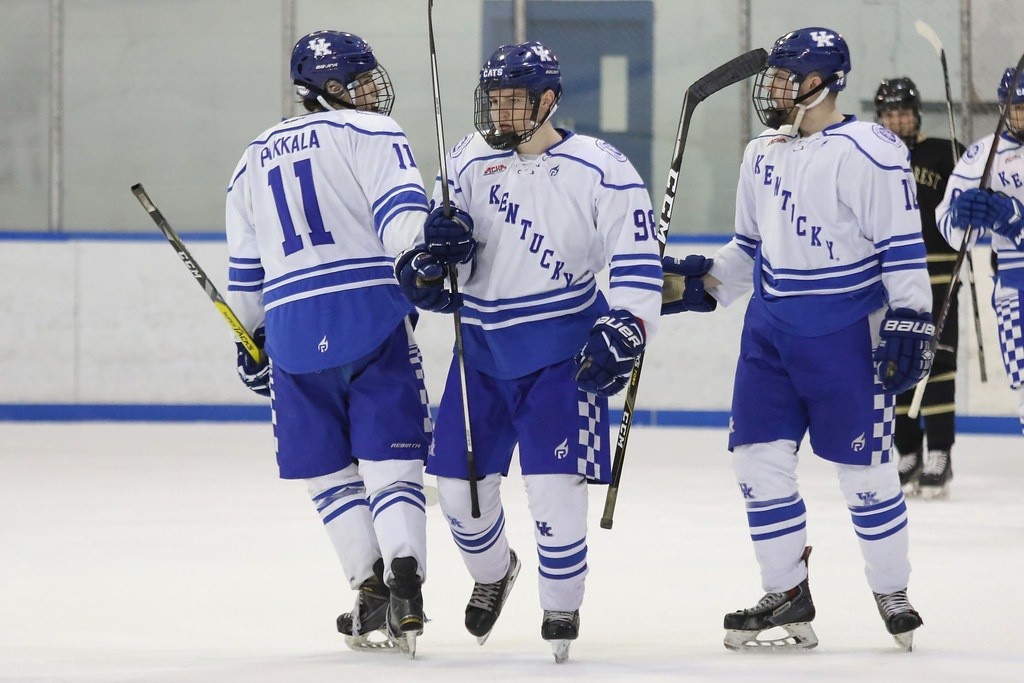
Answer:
[474,40,560,149]
[290,28,396,117]
[997,67,1024,143]
[751,27,852,132]
[874,77,921,139]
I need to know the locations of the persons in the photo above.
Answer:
[426,40,664,664]
[660,27,937,654]
[936,66,1024,438]
[224,30,453,660]
[874,77,967,501]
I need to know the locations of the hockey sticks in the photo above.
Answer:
[130,182,269,366]
[906,52,1024,421]
[422,0,484,521]
[598,46,771,532]
[914,17,989,387]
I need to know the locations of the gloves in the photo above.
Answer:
[873,309,935,392]
[398,254,461,313]
[573,309,645,397]
[950,188,990,228]
[235,325,273,396]
[423,205,475,263]
[982,190,1024,250]
[656,250,716,312]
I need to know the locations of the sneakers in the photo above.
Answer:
[464,548,521,645]
[873,587,922,652]
[542,607,581,663]
[723,545,819,648]
[896,452,922,494]
[337,556,403,653]
[387,556,424,657]
[919,450,954,497]
